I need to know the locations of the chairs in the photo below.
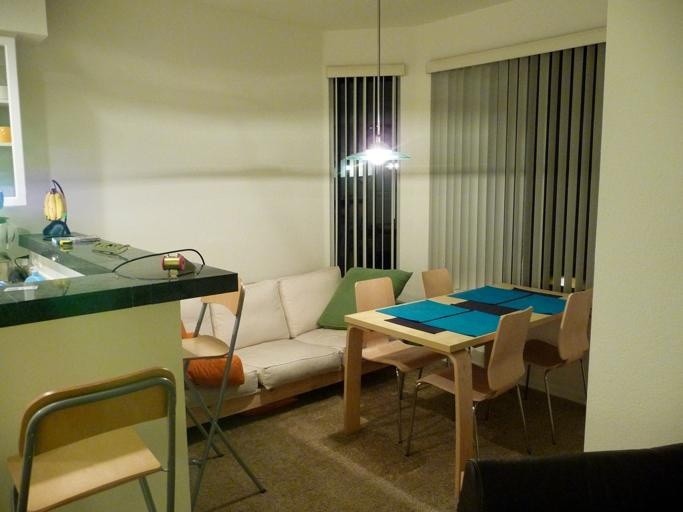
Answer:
[457,444,683,512]
[181,278,265,511]
[405,305,532,460]
[423,267,453,298]
[355,276,452,443]
[524,290,596,444]
[7,364,176,512]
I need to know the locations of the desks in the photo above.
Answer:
[344,284,570,502]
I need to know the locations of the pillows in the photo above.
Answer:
[317,260,412,328]
[180,318,244,387]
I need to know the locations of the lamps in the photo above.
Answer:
[345,1,411,166]
[43,178,71,237]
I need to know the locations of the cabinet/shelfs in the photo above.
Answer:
[1,36,26,207]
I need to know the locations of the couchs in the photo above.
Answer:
[180,266,394,429]
